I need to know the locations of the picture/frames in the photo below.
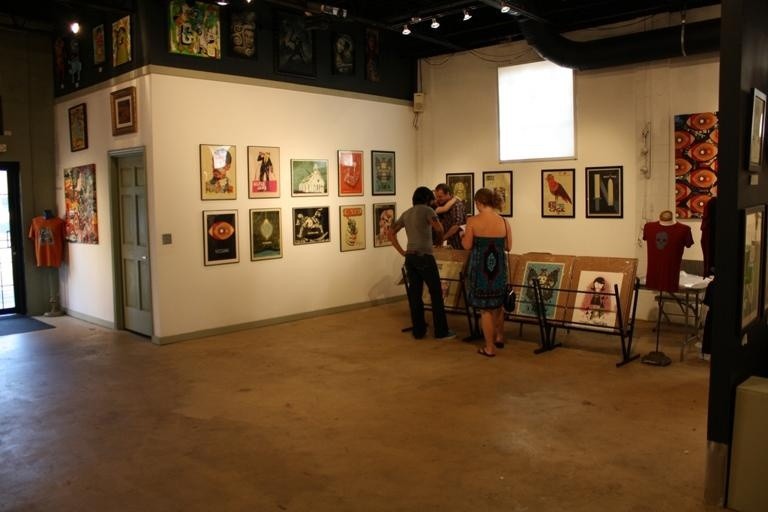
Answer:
[67,102,90,152]
[109,85,139,137]
[198,144,397,267]
[401,247,639,336]
[541,166,624,218]
[740,87,766,336]
[446,170,513,217]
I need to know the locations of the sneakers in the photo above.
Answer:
[436,331,457,340]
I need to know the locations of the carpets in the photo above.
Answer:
[0,313,57,337]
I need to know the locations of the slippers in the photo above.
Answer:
[495,342,504,348]
[478,347,496,357]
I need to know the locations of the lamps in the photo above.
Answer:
[401,2,511,37]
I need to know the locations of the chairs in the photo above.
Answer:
[654,258,702,333]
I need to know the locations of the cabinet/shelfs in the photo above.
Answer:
[401,275,640,368]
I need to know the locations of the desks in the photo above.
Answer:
[633,277,709,362]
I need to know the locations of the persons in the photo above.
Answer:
[387,185,457,341]
[433,183,466,248]
[28,209,66,267]
[640,210,695,292]
[377,206,395,243]
[699,194,717,362]
[207,146,233,196]
[251,148,274,190]
[428,188,462,247]
[457,187,514,357]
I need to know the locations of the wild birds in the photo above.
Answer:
[544,174,573,205]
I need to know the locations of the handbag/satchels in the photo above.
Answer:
[503,289,516,312]
